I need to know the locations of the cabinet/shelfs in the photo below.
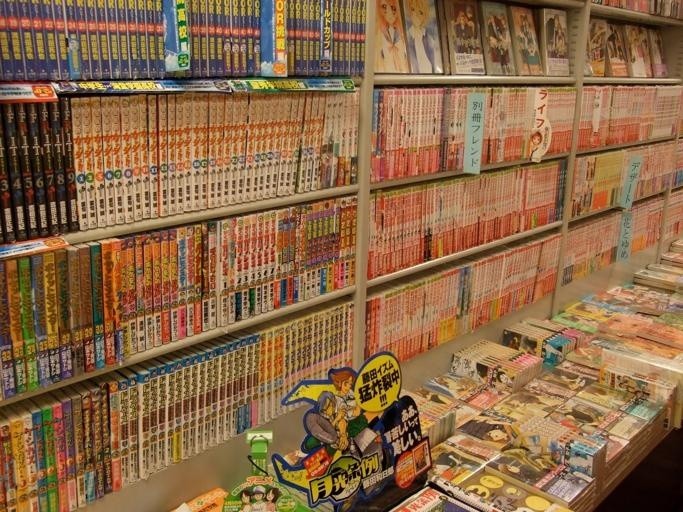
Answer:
[71,238,683,512]
[1,1,683,512]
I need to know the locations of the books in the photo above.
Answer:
[368,158,569,278]
[539,8,571,77]
[604,24,628,77]
[575,85,683,151]
[647,29,670,78]
[505,4,543,76]
[561,195,665,287]
[569,141,676,218]
[663,192,683,240]
[672,143,683,190]
[403,0,445,75]
[588,0,682,21]
[370,87,580,185]
[622,25,653,78]
[373,0,410,75]
[365,228,563,367]
[437,0,485,75]
[478,0,515,77]
[583,18,608,77]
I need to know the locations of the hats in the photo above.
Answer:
[252,486,265,495]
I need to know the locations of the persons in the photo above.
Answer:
[300,367,369,451]
[237,484,280,512]
[404,0,437,72]
[453,4,477,54]
[374,0,406,72]
